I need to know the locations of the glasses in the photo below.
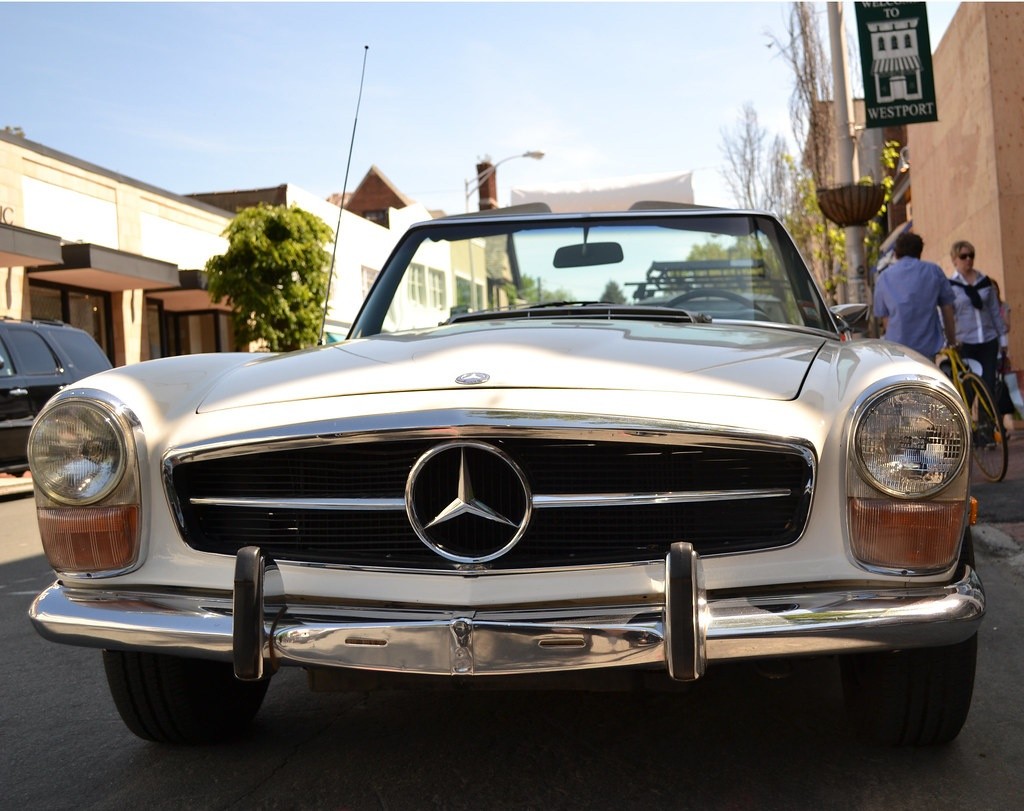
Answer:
[958,253,974,259]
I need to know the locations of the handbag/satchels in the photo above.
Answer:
[993,351,1016,416]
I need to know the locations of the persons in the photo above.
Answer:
[936,239,1009,448]
[989,277,1012,417]
[872,230,957,364]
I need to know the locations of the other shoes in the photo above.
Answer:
[984,443,995,452]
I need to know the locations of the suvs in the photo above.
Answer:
[1,316,114,478]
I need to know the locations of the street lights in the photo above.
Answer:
[465,150,545,313]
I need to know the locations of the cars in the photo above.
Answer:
[26,45,985,746]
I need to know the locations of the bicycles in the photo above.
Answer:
[938,341,1009,482]
